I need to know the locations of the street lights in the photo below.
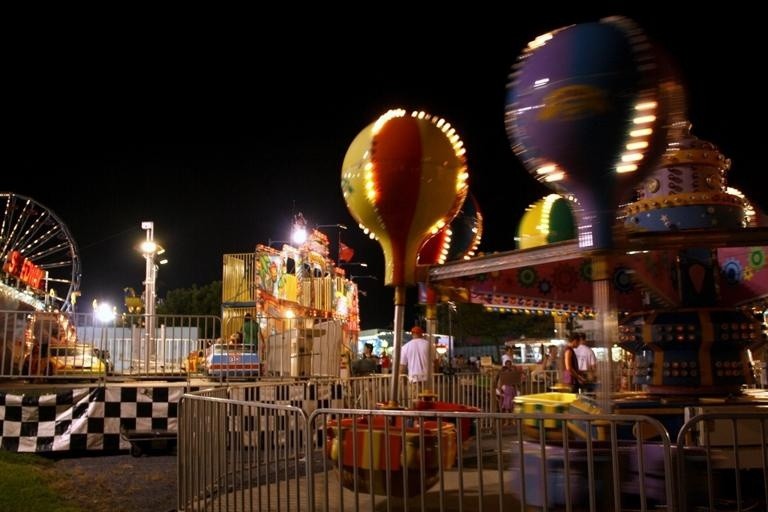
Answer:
[139,220,168,362]
[448,300,456,375]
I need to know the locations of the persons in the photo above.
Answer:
[362,342,378,361]
[398,324,440,393]
[437,353,482,373]
[491,327,597,428]
[240,312,267,352]
[379,351,390,375]
